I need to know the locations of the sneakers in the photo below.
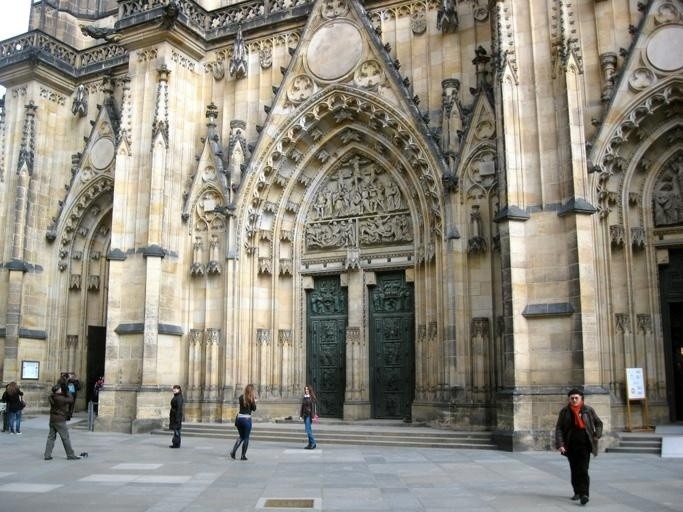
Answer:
[312,415,319,424]
[572,494,589,504]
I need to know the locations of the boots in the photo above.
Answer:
[230,439,242,459]
[241,443,248,460]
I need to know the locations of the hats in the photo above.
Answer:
[568,388,583,397]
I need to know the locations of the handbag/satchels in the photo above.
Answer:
[18,400,26,410]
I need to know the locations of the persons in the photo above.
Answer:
[2,381,23,434]
[556,389,603,505]
[169,386,183,448]
[6,384,10,430]
[43,373,104,460]
[300,384,318,449]
[230,384,256,460]
